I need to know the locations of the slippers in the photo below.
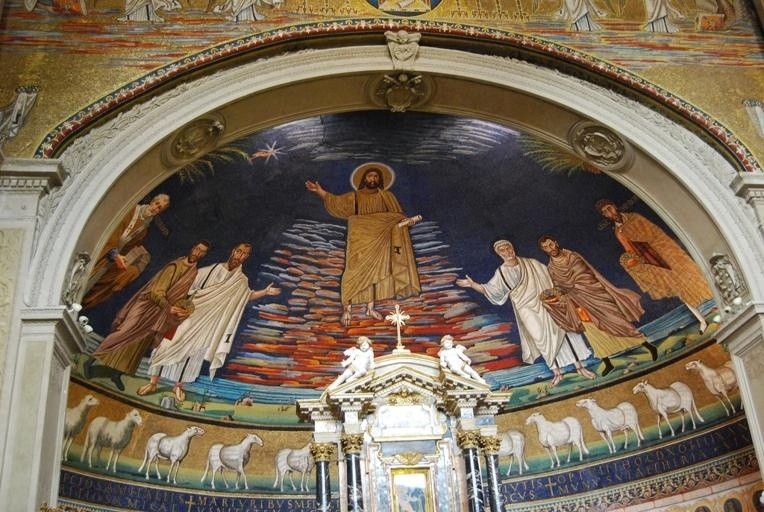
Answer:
[549,375,562,389]
[700,320,709,335]
[137,383,157,396]
[365,309,384,321]
[172,384,186,402]
[340,310,352,328]
[577,368,597,380]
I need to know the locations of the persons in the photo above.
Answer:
[440,335,486,383]
[328,336,375,391]
[305,168,422,326]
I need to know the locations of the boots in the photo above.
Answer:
[83,358,95,379]
[642,341,658,361]
[110,370,125,391]
[602,357,614,377]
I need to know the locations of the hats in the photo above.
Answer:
[493,240,512,252]
